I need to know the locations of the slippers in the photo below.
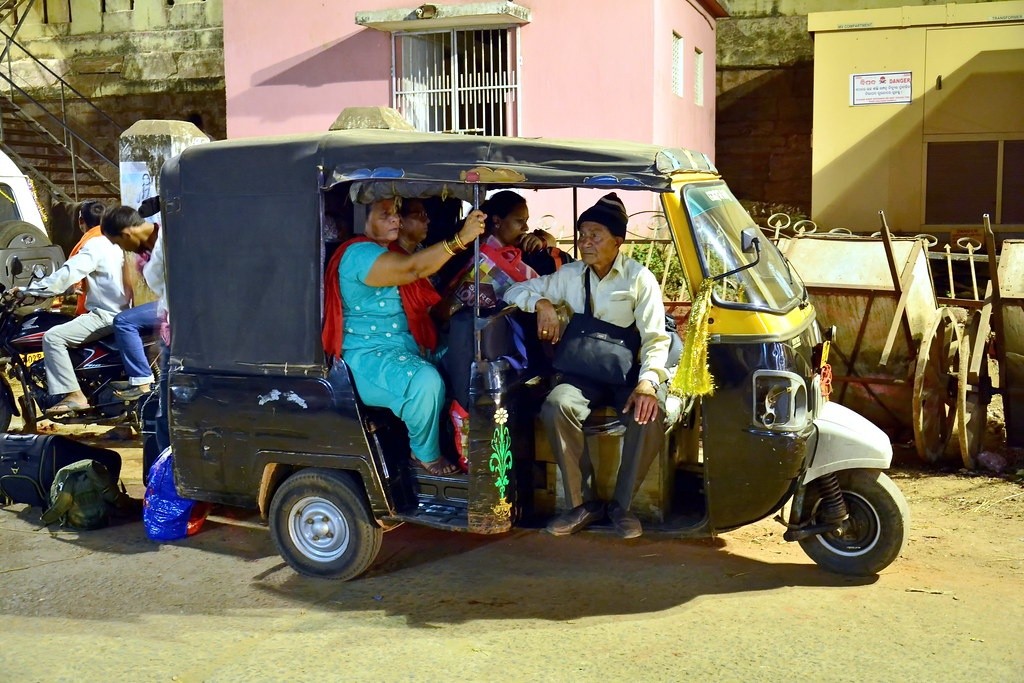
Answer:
[45,401,90,412]
[113,386,152,400]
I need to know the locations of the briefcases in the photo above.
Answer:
[0,433,122,506]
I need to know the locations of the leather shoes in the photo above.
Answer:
[545,501,604,535]
[607,504,643,538]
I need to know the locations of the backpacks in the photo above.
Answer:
[39,459,128,531]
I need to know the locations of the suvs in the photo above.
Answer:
[0,149,65,321]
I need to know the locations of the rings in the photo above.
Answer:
[542,331,548,335]
[479,221,484,228]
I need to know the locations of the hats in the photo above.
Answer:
[576,192,628,241]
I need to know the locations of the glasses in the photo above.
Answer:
[407,210,427,222]
[379,209,399,219]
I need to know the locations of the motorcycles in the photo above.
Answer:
[158,124,912,584]
[0,255,161,436]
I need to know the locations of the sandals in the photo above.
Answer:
[408,452,461,476]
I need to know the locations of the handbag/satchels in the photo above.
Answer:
[550,313,642,409]
[143,446,212,541]
[448,243,536,307]
[522,249,556,276]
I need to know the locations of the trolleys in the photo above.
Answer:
[771,210,963,469]
[954,214,1024,472]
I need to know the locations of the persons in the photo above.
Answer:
[336,192,669,538]
[6,200,172,454]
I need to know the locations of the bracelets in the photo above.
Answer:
[442,233,467,255]
